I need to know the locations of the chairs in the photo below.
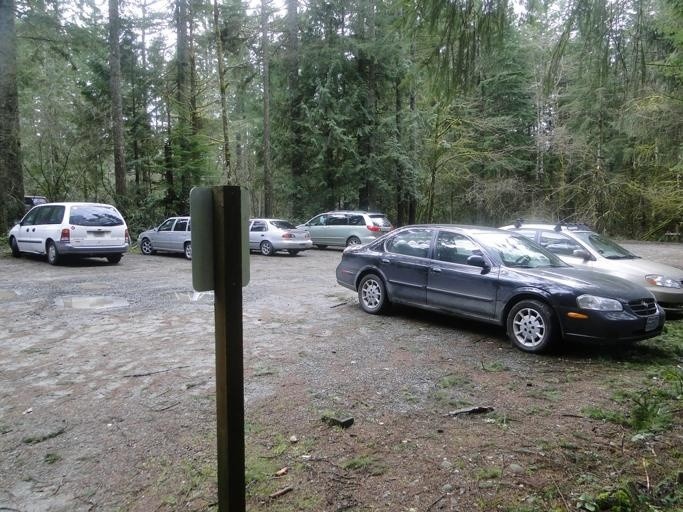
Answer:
[398,243,427,257]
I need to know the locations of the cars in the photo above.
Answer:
[455,221,683,311]
[248,218,313,256]
[137,216,191,260]
[336,223,666,353]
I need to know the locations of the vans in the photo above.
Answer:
[7,195,131,266]
[296,210,394,250]
